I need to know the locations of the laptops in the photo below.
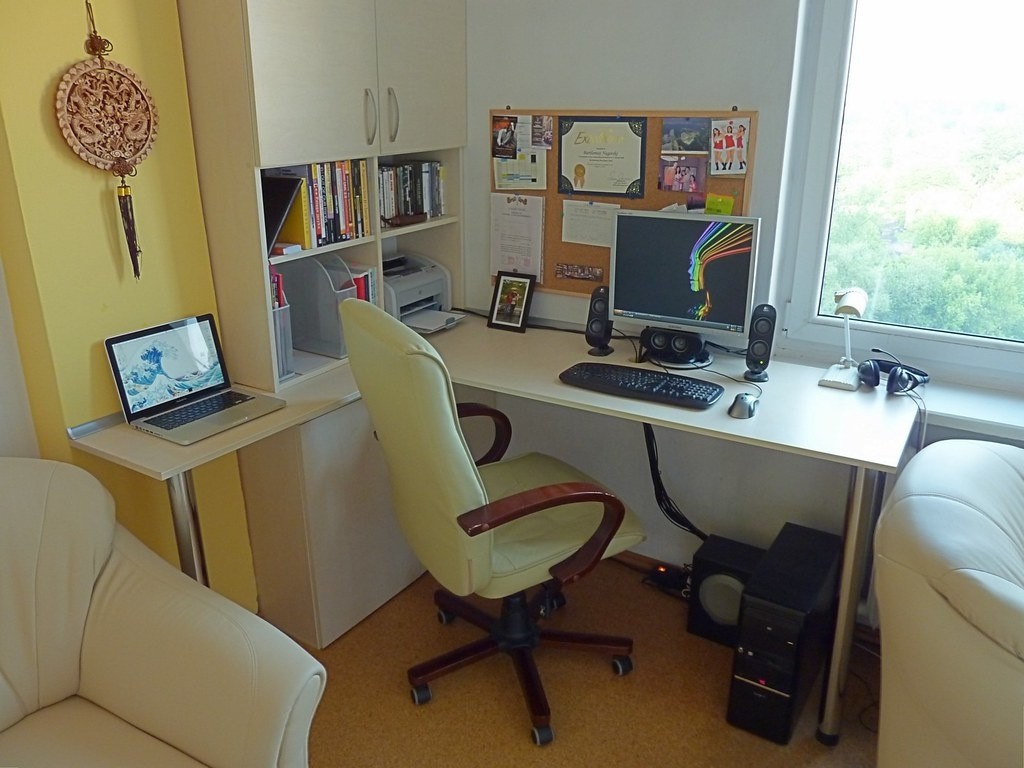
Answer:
[104,313,287,446]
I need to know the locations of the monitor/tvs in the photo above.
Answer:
[609,208,762,369]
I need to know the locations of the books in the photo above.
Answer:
[379,159,445,228]
[263,159,371,250]
[271,274,287,379]
[325,261,379,308]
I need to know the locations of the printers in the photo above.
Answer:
[380,250,469,334]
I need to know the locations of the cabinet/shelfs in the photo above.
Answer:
[176,0,503,392]
[237,392,427,649]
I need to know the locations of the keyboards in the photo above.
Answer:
[559,362,724,407]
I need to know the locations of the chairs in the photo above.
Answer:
[338,297,650,745]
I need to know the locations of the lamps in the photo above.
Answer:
[818,286,869,390]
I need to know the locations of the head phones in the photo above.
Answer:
[858,358,931,394]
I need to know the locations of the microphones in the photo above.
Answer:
[871,348,903,369]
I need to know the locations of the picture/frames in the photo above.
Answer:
[487,270,537,334]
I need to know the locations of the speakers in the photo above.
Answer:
[639,327,707,359]
[687,534,767,648]
[585,286,614,357]
[744,303,776,382]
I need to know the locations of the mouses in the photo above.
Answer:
[727,392,760,419]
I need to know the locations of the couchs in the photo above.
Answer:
[0,456,328,768]
[874,438,1024,768]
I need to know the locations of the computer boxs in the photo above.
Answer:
[725,521,846,745]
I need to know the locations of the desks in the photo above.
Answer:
[66,309,926,746]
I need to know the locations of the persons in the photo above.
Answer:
[712,127,726,170]
[503,289,518,315]
[722,126,737,170]
[662,161,697,193]
[732,124,747,170]
